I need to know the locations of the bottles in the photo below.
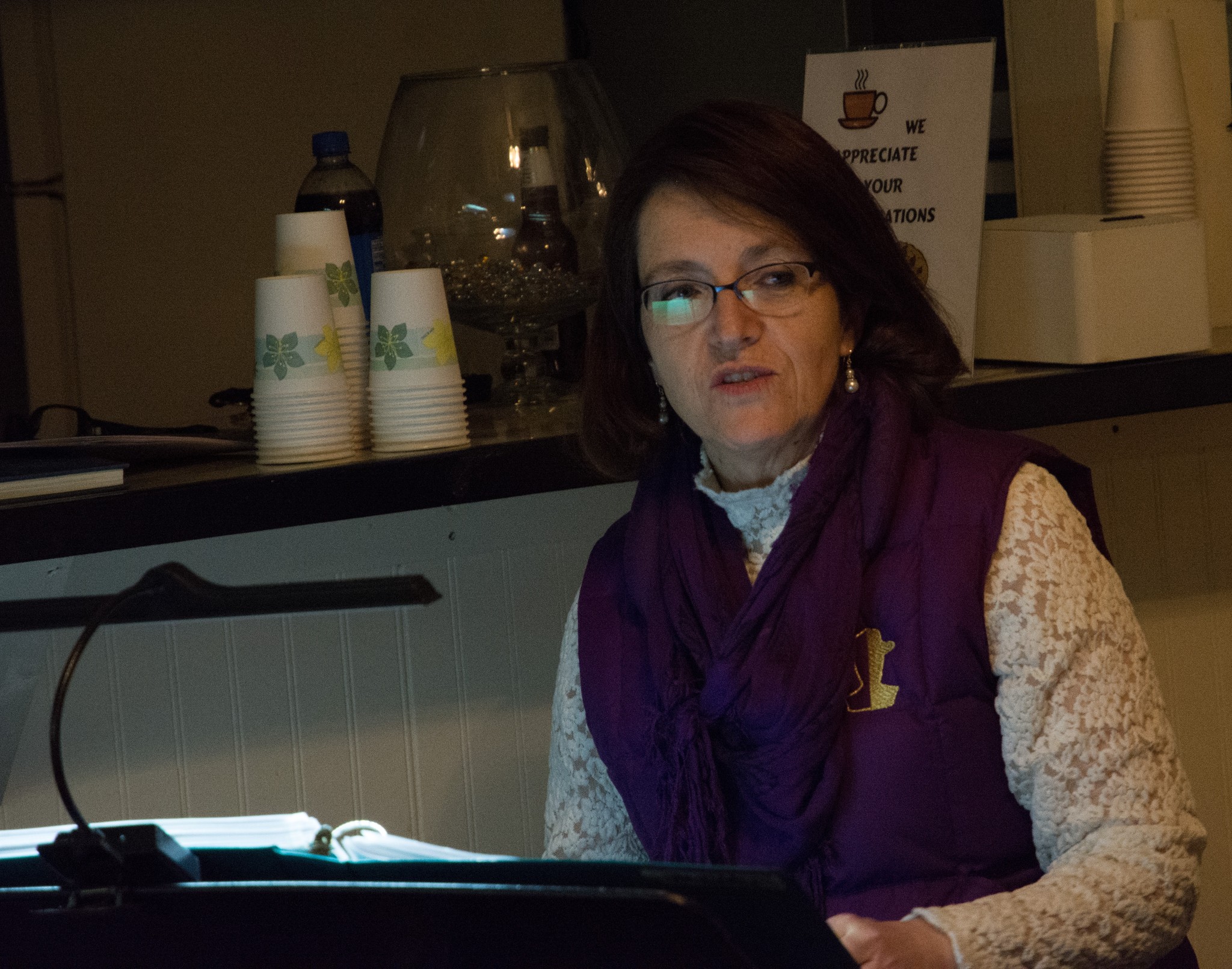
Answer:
[508,122,590,397]
[293,132,386,343]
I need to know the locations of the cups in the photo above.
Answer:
[250,202,473,466]
[1096,16,1201,231]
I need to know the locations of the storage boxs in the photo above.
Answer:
[972,212,1210,364]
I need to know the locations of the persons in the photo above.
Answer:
[537,111,1211,967]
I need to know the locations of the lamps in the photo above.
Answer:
[0,562,444,876]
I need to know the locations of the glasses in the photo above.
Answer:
[638,260,817,325]
